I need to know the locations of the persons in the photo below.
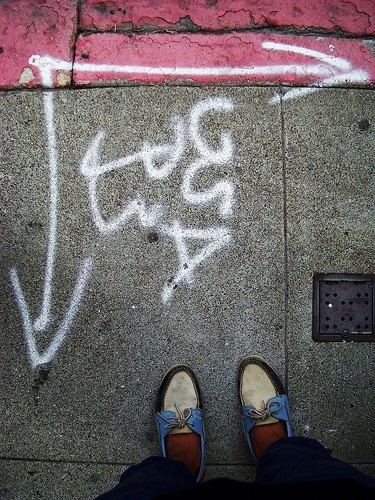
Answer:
[94,355,375,500]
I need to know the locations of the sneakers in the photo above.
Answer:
[236,355,292,464]
[154,364,206,483]
